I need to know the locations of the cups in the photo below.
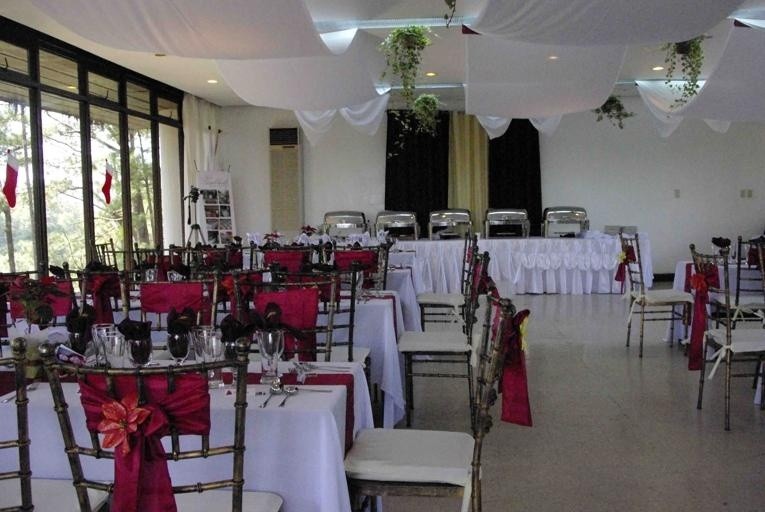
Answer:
[91,324,286,389]
[144,268,158,282]
[350,270,364,291]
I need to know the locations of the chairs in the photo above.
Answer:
[618,229,765,431]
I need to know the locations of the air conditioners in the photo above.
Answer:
[268,127,305,232]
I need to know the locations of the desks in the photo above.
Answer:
[296,230,654,295]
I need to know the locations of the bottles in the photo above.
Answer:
[261,267,273,282]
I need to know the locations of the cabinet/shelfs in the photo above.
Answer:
[198,187,236,248]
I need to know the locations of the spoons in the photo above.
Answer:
[278,386,299,408]
[260,383,284,408]
